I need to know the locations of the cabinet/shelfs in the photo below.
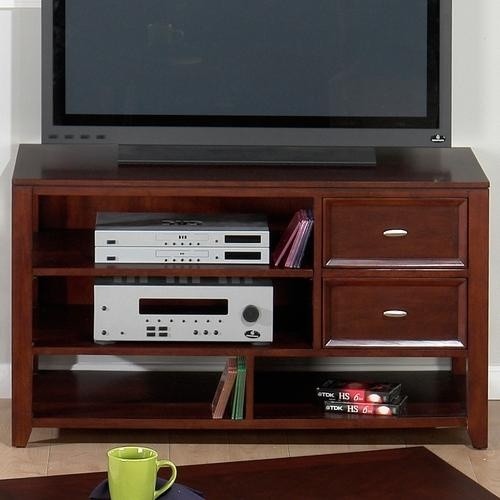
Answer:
[313,148,490,358]
[11,144,491,451]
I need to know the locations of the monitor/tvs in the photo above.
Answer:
[41,2,452,166]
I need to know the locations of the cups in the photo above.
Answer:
[107,446,177,500]
